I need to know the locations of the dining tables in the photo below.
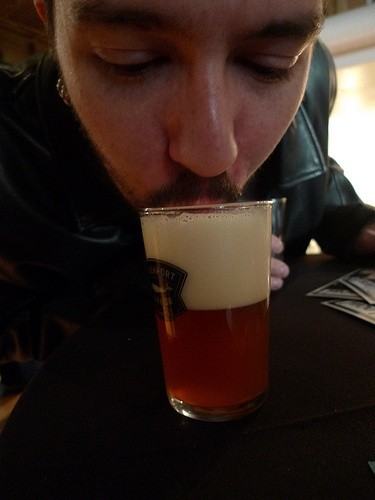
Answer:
[1,253,375,500]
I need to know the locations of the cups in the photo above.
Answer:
[136,199,273,421]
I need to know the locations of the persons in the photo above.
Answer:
[0,0,375,391]
